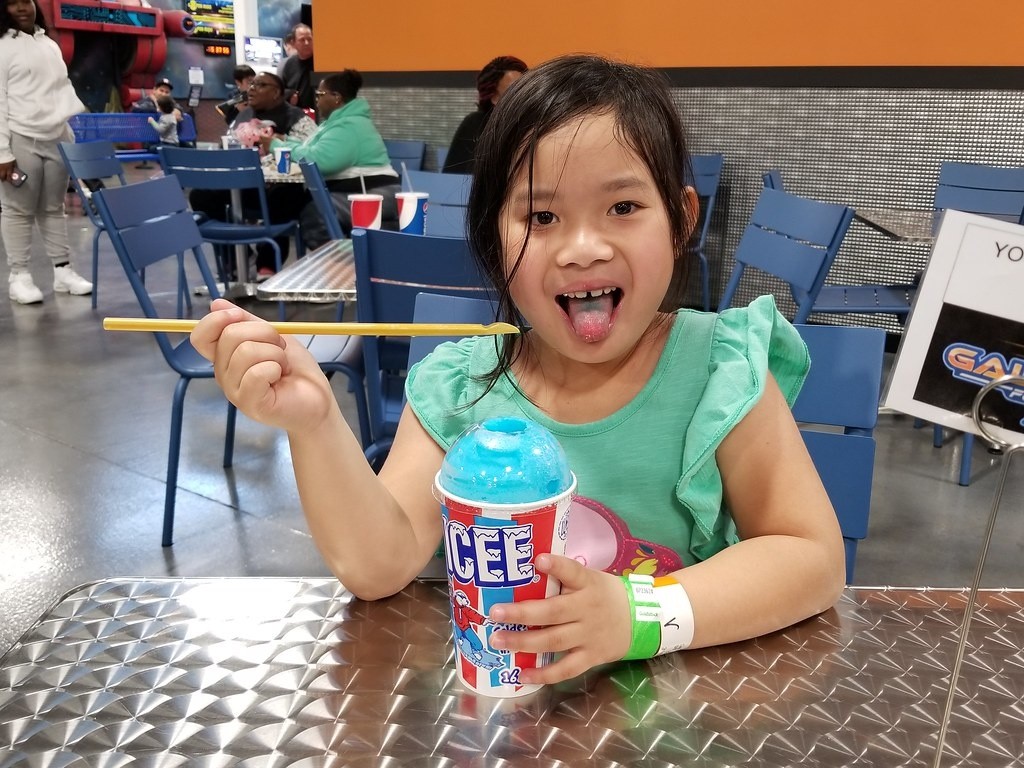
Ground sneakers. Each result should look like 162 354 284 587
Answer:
53 264 93 295
9 272 43 305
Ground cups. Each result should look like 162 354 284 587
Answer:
274 148 292 174
222 135 233 150
347 194 383 230
432 419 577 697
394 192 429 236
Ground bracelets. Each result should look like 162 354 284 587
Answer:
619 572 661 661
652 575 695 658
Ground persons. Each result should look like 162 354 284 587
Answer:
276 21 316 111
258 68 402 251
0 0 95 304
66 55 107 217
188 51 849 683
225 64 256 124
130 78 185 113
146 95 180 147
188 72 314 284
441 55 528 174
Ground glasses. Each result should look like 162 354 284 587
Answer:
248 82 279 89
314 90 336 98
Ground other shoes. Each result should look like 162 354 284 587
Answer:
83 198 98 215
256 268 275 281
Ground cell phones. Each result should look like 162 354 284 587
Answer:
9 166 28 187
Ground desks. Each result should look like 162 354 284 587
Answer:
854 209 1024 455
169 164 309 298
1 575 1024 768
258 236 358 302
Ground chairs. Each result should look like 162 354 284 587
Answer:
57 137 1023 586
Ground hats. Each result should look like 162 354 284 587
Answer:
156 78 174 91
